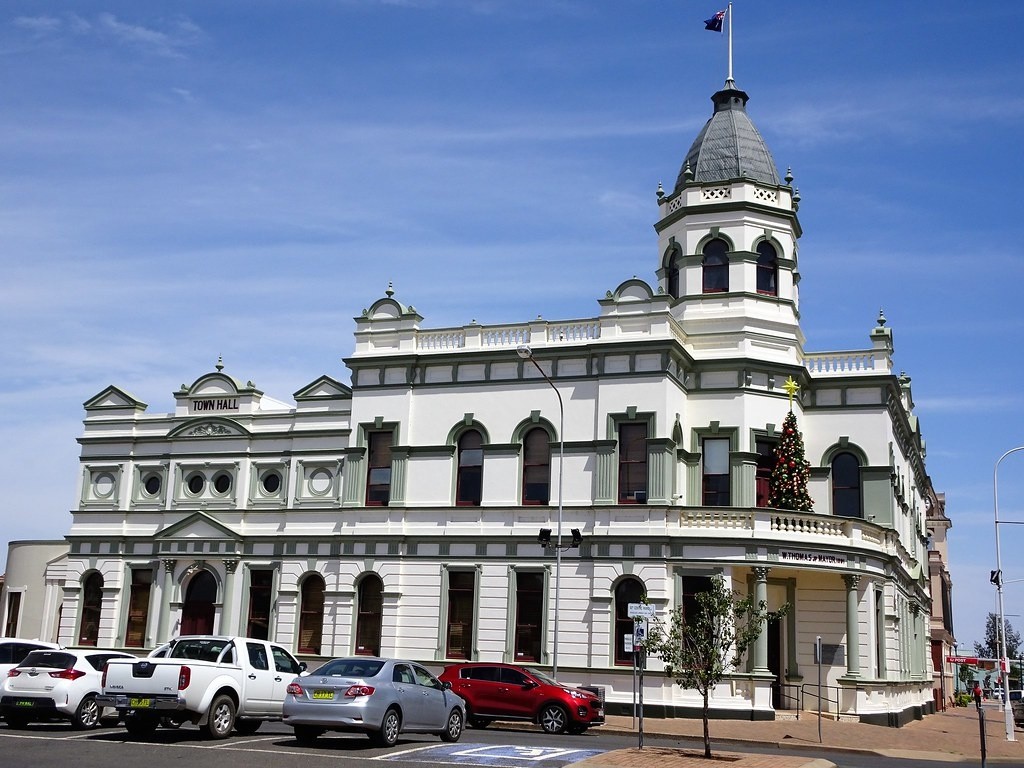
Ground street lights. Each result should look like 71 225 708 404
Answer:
516 343 564 682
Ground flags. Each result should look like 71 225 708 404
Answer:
703 9 726 32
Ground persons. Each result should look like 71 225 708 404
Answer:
972 682 982 712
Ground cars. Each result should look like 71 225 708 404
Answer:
0 649 142 731
0 637 69 686
282 655 467 748
994 688 1006 699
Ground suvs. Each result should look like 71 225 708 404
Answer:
437 661 606 734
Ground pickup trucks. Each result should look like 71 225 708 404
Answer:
93 636 309 739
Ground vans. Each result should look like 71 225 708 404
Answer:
1009 690 1024 709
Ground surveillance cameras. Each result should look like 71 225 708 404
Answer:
868 515 875 518
673 494 682 499
